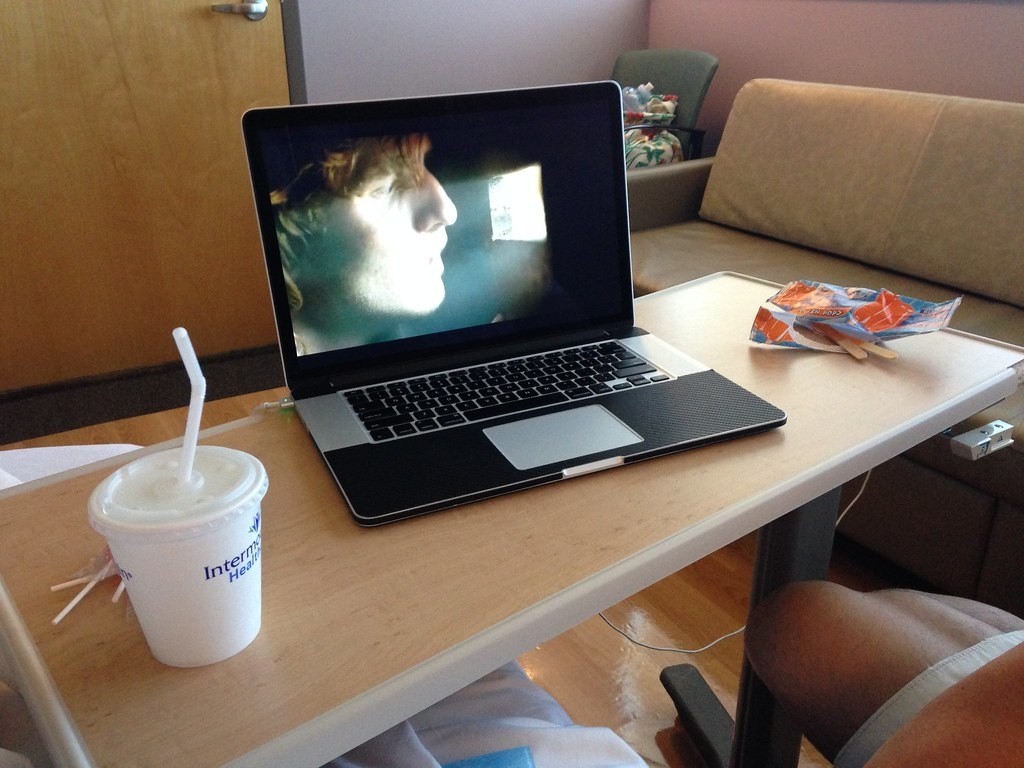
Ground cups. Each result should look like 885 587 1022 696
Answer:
88 444 269 667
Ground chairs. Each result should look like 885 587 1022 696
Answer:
612 49 718 167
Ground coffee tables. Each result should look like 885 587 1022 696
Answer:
3 269 1024 766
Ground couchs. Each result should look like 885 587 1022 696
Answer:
627 77 1024 620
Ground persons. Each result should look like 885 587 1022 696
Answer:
745 570 1024 767
265 113 459 356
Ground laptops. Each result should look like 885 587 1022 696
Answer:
240 77 788 531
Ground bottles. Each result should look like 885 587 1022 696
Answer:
622 82 654 113
